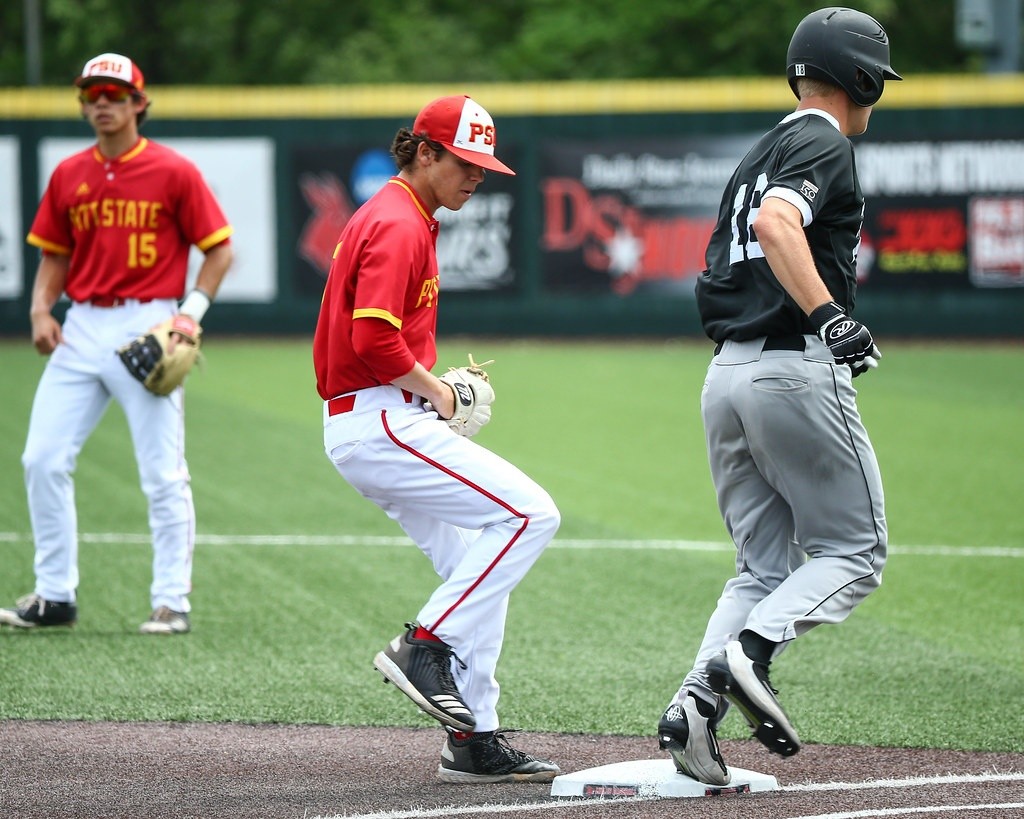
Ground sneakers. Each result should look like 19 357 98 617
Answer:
373 621 476 733
139 605 190 635
657 687 731 786
0 592 76 628
439 726 561 784
706 633 800 759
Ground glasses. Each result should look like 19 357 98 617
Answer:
81 83 135 104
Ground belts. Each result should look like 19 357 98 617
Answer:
714 335 806 351
328 388 412 416
91 295 153 307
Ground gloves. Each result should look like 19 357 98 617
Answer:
810 302 883 378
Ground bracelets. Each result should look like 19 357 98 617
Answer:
180 292 209 322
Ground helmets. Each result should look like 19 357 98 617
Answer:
787 7 904 107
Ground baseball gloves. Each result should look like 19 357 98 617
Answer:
429 351 498 438
115 313 203 407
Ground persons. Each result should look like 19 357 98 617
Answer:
657 7 902 786
313 95 560 779
1 54 235 634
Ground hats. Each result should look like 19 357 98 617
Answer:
413 95 516 177
76 53 143 94
